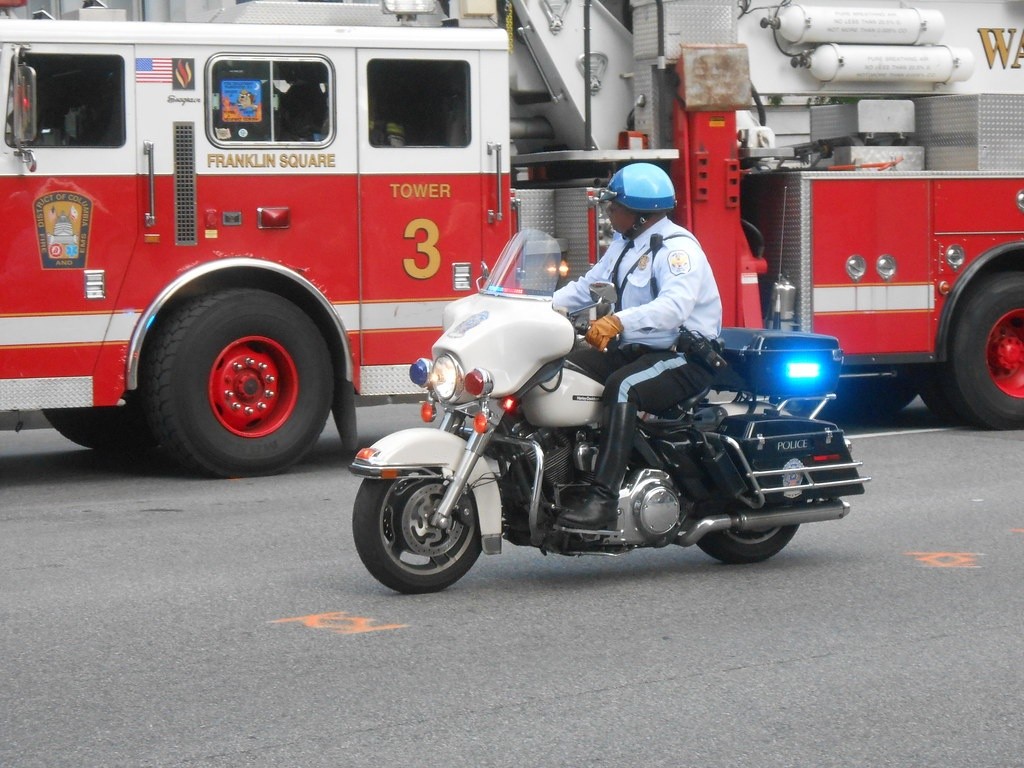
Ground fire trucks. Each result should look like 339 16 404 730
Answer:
0 0 1024 477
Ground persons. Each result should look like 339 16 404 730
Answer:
370 60 466 148
273 64 327 142
552 162 723 533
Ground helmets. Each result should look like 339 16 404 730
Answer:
598 162 677 212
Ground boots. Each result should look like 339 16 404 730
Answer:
556 402 639 530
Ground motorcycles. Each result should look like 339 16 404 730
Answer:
349 229 871 595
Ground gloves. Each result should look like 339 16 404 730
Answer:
587 315 623 352
